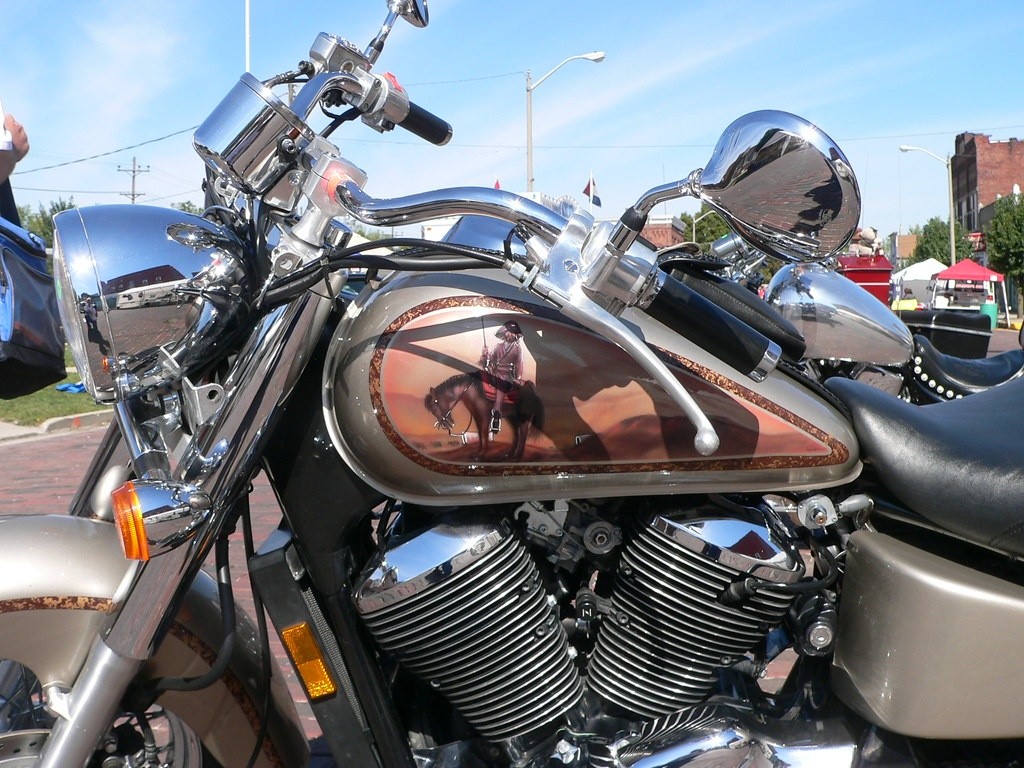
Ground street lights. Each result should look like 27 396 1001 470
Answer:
526 51 606 194
898 144 956 267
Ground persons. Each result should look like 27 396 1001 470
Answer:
80 297 98 330
904 288 914 299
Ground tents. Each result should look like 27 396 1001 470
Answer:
926 259 1015 329
890 257 953 310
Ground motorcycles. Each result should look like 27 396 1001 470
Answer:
1 0 1022 768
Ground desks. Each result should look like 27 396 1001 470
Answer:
931 306 981 311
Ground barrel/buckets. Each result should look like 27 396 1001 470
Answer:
979 303 998 329
